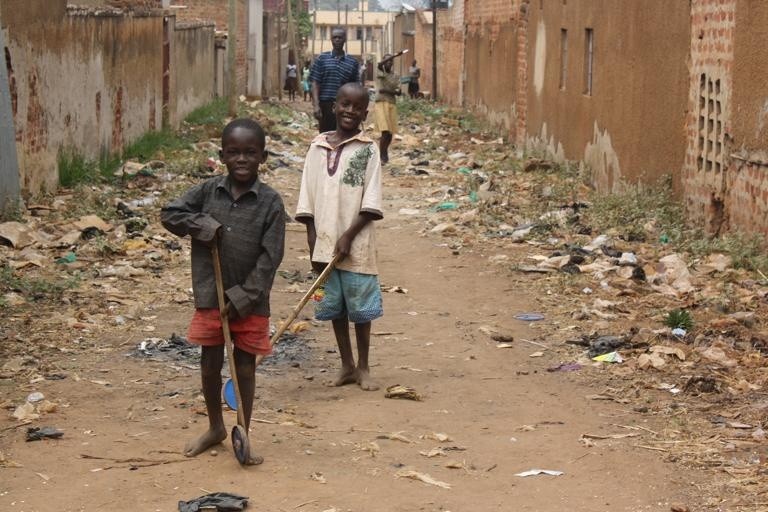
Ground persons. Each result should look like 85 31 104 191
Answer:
281 51 422 101
312 26 360 136
295 83 404 391
158 119 288 466
375 49 410 162
